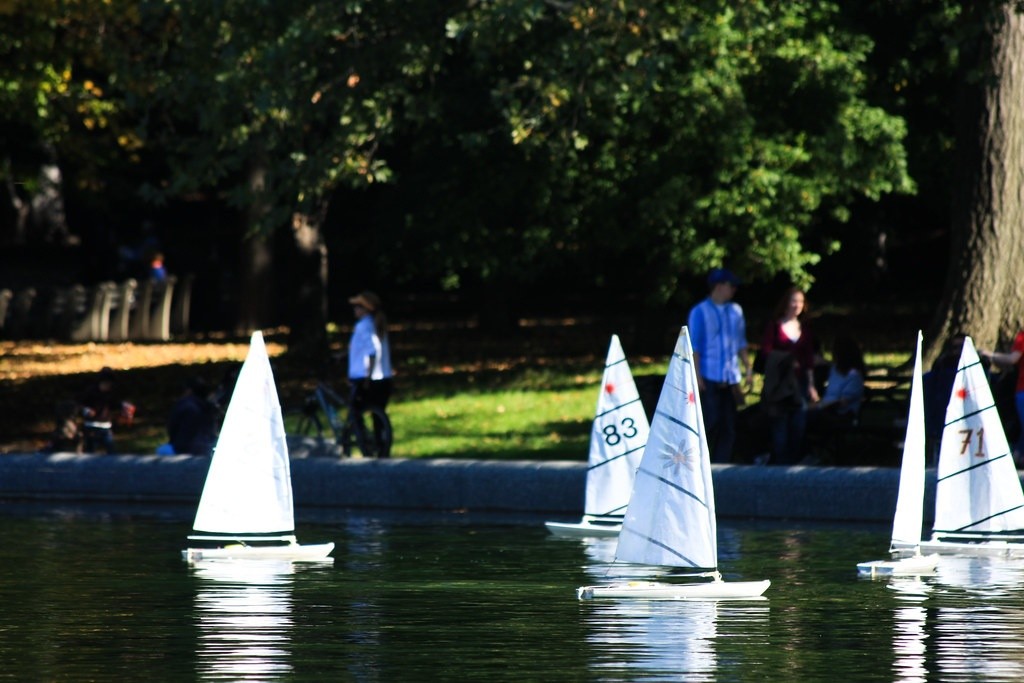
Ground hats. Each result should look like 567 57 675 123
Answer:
710 270 742 286
349 295 374 311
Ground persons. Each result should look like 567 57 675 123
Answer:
688 271 753 464
341 291 395 457
809 337 863 457
751 287 821 464
167 384 212 456
979 328 1024 465
43 376 136 454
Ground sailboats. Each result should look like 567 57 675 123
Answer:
179 328 337 568
573 324 773 605
544 332 651 539
855 329 1024 588
